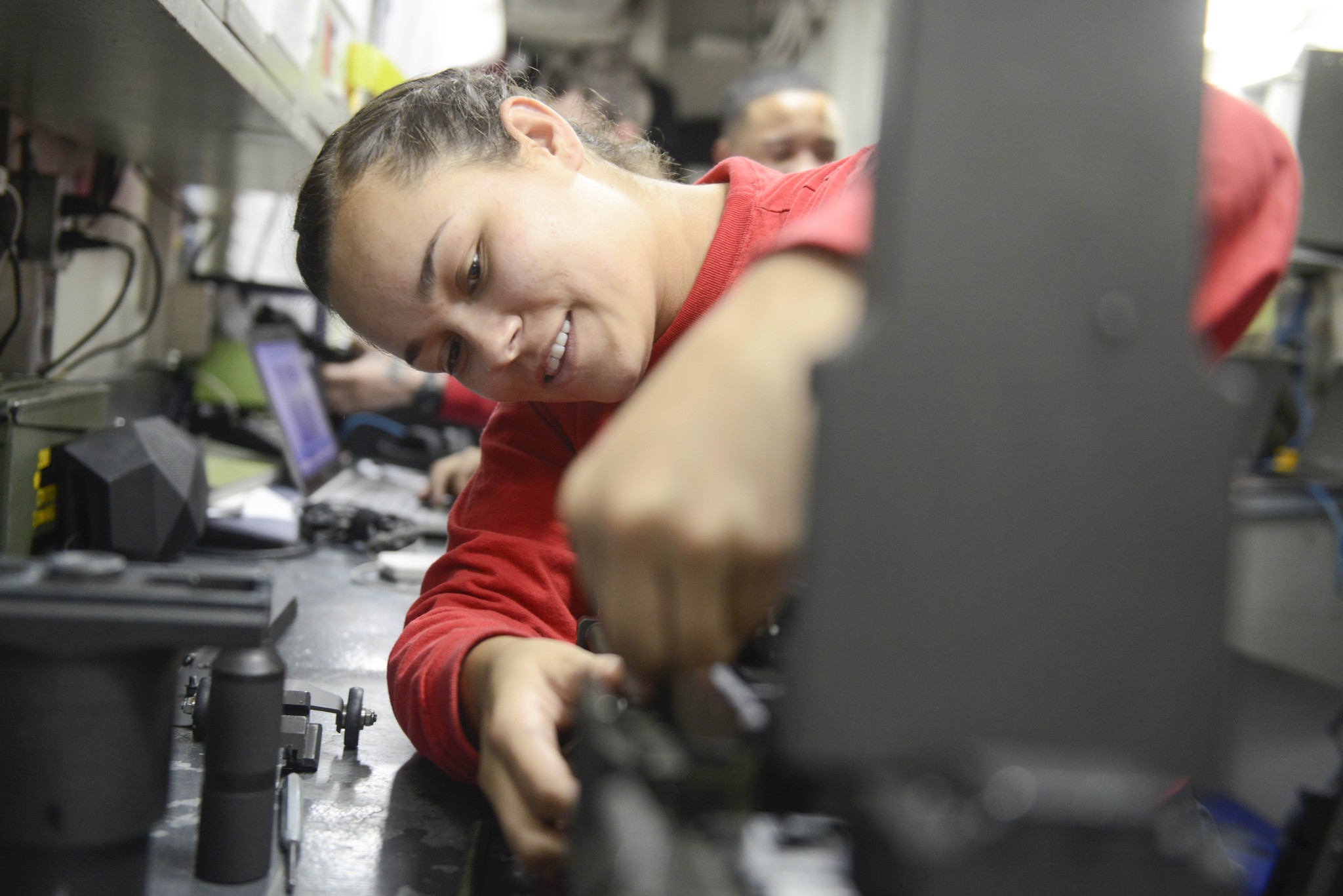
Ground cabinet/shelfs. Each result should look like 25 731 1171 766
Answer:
0 1 482 199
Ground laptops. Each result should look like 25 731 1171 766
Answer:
249 325 458 535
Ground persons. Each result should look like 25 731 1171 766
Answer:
322 46 658 507
712 64 845 176
295 59 1302 896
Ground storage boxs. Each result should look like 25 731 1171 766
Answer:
247 1 507 124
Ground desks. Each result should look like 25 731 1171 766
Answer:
0 533 466 896
